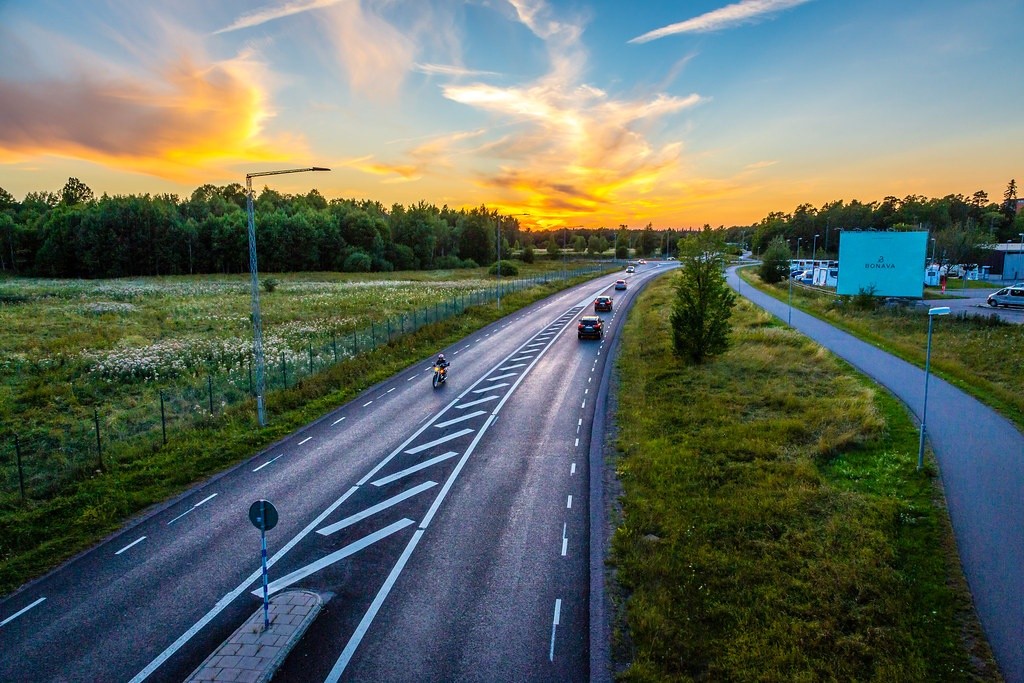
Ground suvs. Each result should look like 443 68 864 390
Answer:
578 316 605 340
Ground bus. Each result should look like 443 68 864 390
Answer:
793 259 834 271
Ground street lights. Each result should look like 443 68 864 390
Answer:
930 238 936 292
243 167 333 425
915 307 951 473
494 213 529 309
797 237 803 275
1004 240 1012 283
812 234 820 286
757 246 760 263
1018 232 1024 283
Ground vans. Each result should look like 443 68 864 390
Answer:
987 287 1024 307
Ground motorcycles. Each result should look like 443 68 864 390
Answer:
431 361 451 388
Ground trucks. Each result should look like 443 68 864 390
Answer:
813 267 838 287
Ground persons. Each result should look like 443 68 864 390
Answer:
433 354 446 378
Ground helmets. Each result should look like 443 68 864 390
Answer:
439 354 444 360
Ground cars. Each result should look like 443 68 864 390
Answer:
639 259 646 263
625 266 635 272
667 257 674 260
789 269 812 286
594 296 613 311
614 280 628 289
931 258 991 281
